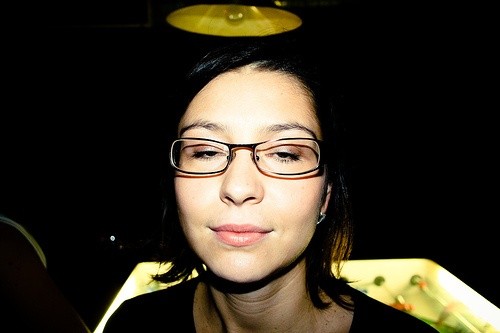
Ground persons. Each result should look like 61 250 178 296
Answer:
103 43 438 333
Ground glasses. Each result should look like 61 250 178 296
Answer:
169 138 327 176
